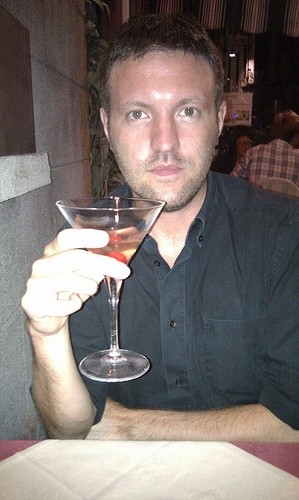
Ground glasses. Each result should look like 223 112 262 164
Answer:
238 141 251 144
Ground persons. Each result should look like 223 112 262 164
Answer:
20 11 299 441
218 134 257 176
230 108 299 187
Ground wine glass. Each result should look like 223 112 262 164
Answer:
56 197 166 382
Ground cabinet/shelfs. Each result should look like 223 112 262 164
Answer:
206 24 298 153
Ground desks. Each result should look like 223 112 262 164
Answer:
0 441 298 500
